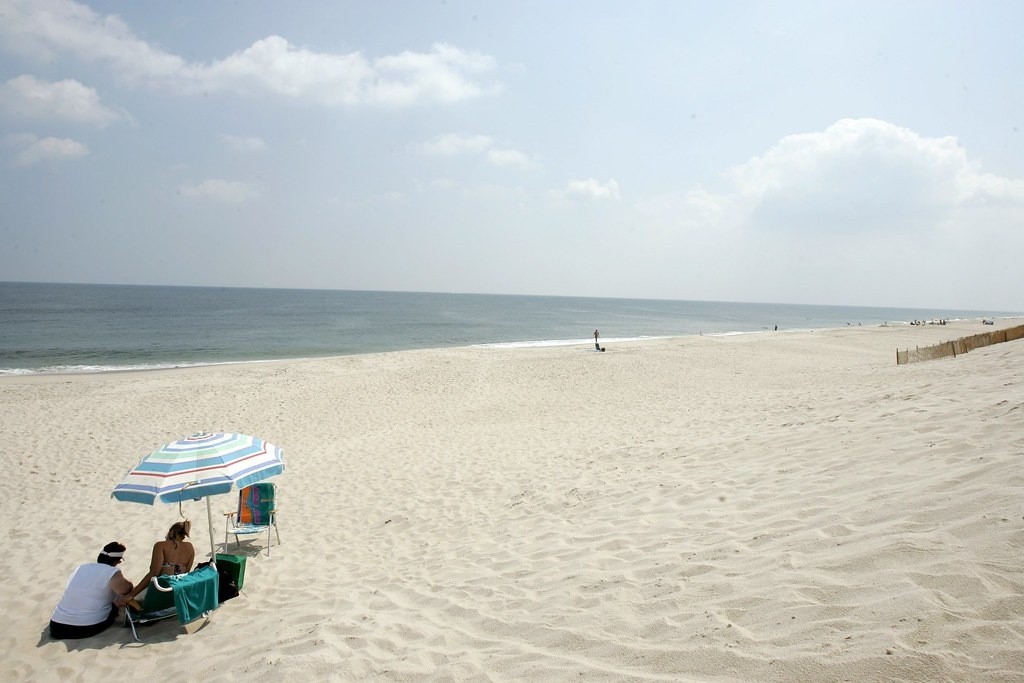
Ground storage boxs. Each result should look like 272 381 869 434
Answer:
209 553 247 591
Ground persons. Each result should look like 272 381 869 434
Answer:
593 329 600 342
983 319 993 325
910 318 945 326
113 520 195 608
50 541 133 639
775 323 778 331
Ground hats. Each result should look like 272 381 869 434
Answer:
100 550 125 561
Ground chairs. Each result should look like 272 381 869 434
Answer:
111 562 219 641
224 482 281 557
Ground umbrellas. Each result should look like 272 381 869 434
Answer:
109 431 287 565
